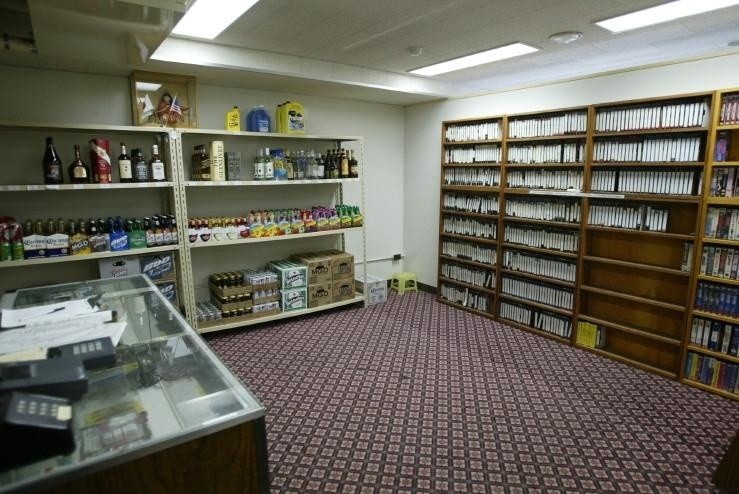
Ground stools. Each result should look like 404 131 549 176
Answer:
391 271 418 296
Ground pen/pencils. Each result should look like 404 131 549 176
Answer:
19 307 65 322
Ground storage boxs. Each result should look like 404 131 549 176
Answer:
98 251 180 314
355 273 388 305
267 249 355 312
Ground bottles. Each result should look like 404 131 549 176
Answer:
313 204 360 218
192 145 210 181
246 105 270 132
1 222 24 260
248 207 313 223
118 139 166 181
189 215 249 228
225 104 240 130
24 216 143 236
42 137 63 185
144 212 178 247
68 144 90 184
254 145 359 180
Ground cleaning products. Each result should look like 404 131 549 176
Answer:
226 105 240 131
277 101 305 135
245 105 270 133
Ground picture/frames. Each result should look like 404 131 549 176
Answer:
129 69 199 130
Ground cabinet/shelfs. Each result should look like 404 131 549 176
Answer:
679 88 738 398
175 127 369 337
0 274 273 493
573 92 717 384
0 120 192 348
506 105 591 349
439 114 507 320
